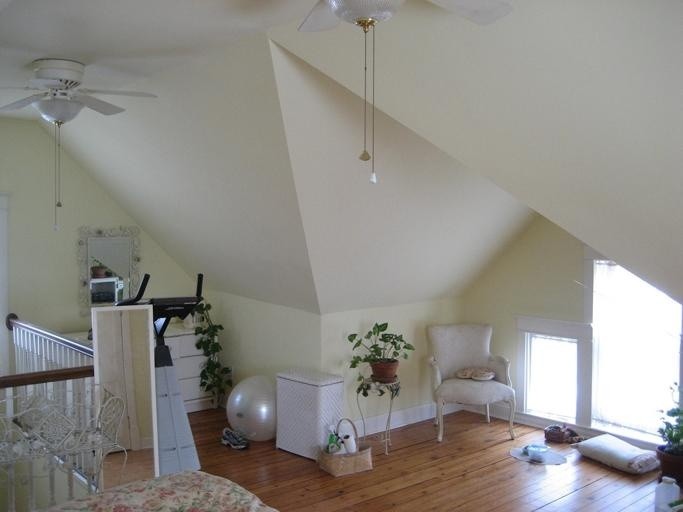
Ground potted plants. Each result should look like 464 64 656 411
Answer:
342 321 413 382
653 385 683 483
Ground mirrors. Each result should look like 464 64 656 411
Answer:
76 226 142 318
92 304 159 489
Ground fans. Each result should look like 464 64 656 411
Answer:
0 58 158 114
293 0 515 34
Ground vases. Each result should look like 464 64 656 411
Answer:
91 266 108 278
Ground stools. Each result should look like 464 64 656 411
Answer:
275 368 345 463
356 379 402 457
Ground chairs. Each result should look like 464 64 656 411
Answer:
423 320 516 444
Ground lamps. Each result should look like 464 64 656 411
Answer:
328 1 402 33
31 90 85 127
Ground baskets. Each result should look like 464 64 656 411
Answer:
318 418 373 477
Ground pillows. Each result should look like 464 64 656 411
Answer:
457 365 497 382
572 434 659 478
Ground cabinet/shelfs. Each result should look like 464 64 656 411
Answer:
162 320 219 413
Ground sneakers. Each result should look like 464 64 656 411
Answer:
220 429 247 449
